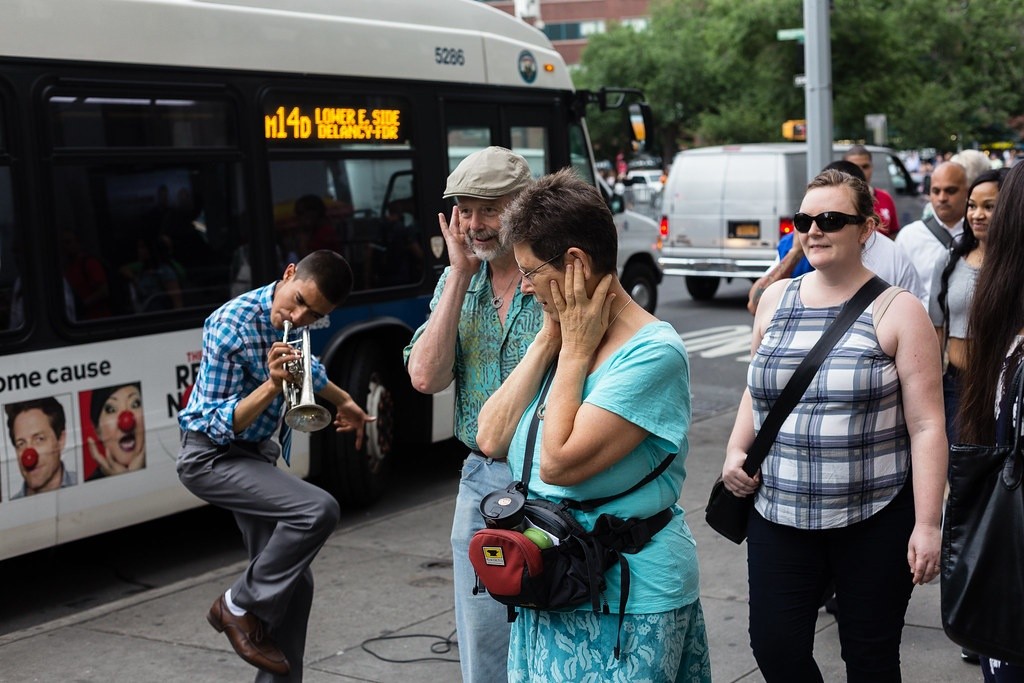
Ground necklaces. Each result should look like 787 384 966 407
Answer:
489 267 521 309
537 300 631 420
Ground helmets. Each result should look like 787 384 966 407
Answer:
443 145 532 203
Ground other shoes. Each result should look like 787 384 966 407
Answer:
962 649 976 666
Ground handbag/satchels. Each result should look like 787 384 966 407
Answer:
705 475 753 545
937 362 1024 661
467 481 674 614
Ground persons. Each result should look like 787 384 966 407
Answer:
5 397 76 498
176 251 376 683
747 147 928 316
84 381 146 482
922 149 1024 683
895 149 989 292
403 146 544 683
56 194 425 324
476 169 713 682
721 169 949 683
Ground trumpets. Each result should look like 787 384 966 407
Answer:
281 320 331 432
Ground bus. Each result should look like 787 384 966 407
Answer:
0 0 656 566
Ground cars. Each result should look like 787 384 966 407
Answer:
625 169 667 210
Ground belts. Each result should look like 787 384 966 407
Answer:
471 448 507 462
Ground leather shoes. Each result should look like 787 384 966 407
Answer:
206 588 290 676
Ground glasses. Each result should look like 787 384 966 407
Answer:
795 211 865 236
518 250 570 281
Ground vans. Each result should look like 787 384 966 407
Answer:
655 140 932 296
329 145 666 318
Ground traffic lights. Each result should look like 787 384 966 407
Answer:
783 120 808 141
